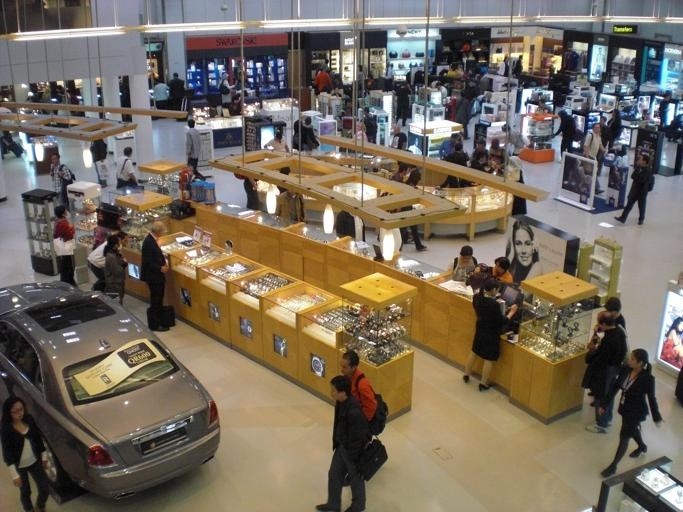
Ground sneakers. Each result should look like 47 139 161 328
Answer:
629 446 647 457
585 423 609 433
594 189 604 194
415 245 424 249
316 503 340 511
343 506 365 512
614 216 626 223
600 464 616 476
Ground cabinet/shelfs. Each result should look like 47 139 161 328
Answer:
594 456 683 511
17 86 682 427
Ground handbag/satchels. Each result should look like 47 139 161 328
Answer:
146 305 175 327
356 439 387 481
115 178 137 189
647 175 654 192
368 394 388 436
52 237 76 256
572 129 584 142
579 136 584 154
40 448 56 482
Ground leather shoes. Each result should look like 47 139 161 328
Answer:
151 324 168 331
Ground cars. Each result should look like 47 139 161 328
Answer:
0 279 221 503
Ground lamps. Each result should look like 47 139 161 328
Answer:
0 1 136 170
208 2 466 259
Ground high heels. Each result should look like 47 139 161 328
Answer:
478 383 489 391
462 374 469 383
